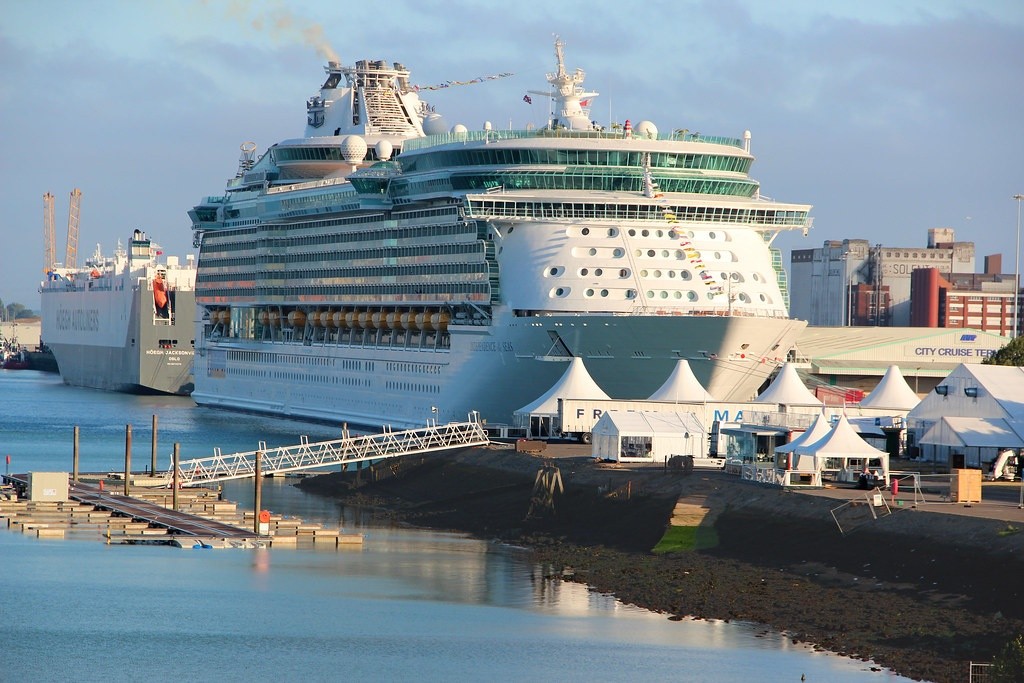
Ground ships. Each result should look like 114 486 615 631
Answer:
187 32 817 445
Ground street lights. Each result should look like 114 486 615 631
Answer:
1012 194 1024 340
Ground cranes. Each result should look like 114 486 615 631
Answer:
41 188 82 281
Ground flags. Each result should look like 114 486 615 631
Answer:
580 98 593 107
523 96 532 104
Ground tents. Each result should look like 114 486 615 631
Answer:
514 358 1024 489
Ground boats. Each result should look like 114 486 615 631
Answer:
319 312 334 328
358 311 374 330
430 312 449 330
209 311 218 323
345 312 360 330
385 312 402 331
307 310 322 327
288 310 307 327
415 312 433 331
400 312 418 330
332 310 345 328
217 311 230 324
371 311 388 331
258 311 270 326
37 227 197 396
153 276 167 309
269 311 281 328
